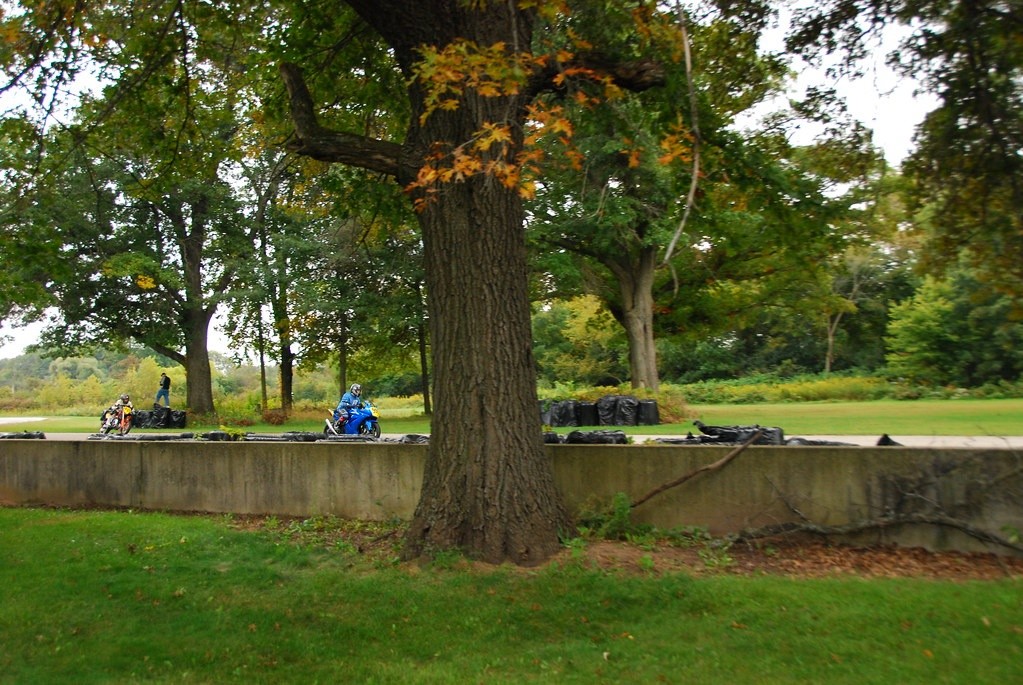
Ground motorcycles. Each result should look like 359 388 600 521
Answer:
100 404 136 434
324 400 381 438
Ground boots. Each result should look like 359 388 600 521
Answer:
333 419 340 428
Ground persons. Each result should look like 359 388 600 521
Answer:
156 373 170 406
104 394 135 427
333 383 362 432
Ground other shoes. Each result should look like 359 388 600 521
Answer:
164 406 169 408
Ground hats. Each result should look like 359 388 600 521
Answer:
161 373 165 376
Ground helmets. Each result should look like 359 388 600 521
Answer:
120 394 129 404
350 384 361 397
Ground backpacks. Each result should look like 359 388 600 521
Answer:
163 376 170 389
